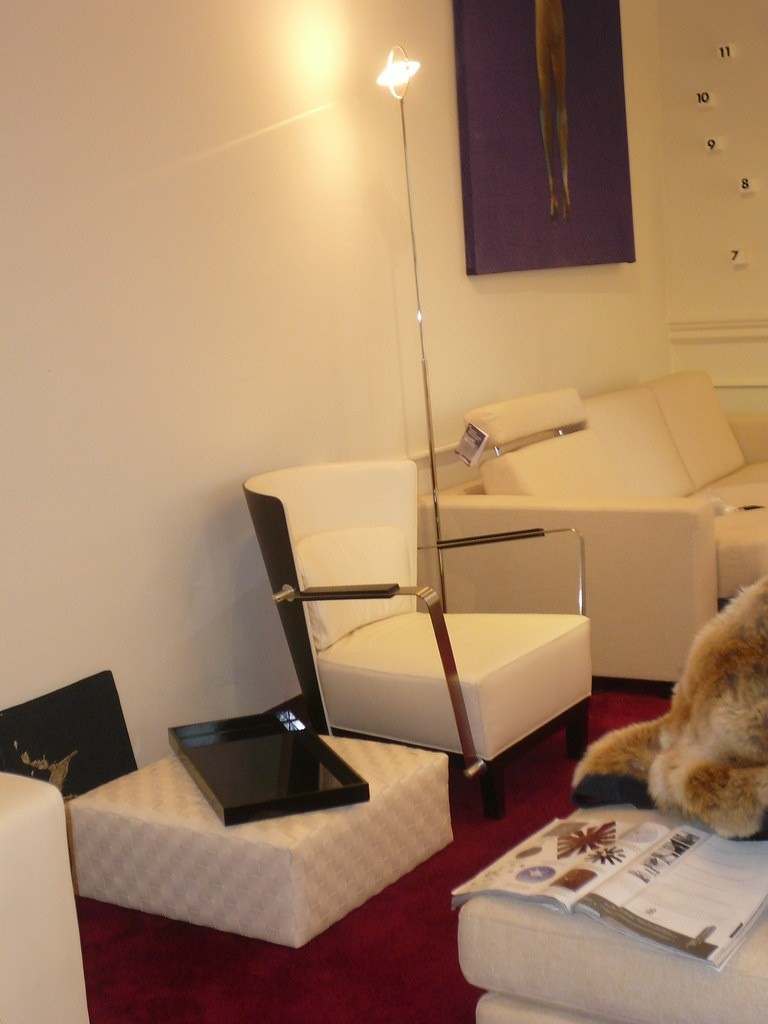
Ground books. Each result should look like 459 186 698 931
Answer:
449 814 768 970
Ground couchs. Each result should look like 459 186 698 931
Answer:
422 367 768 685
455 805 768 1024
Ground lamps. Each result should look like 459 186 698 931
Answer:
376 44 447 614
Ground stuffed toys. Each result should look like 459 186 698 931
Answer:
569 576 767 838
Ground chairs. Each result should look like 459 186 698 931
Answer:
242 458 595 821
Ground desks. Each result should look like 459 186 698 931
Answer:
65 730 458 949
